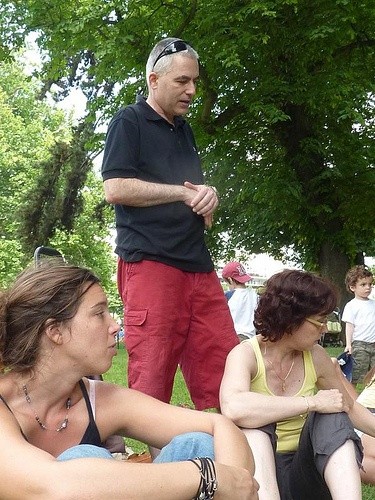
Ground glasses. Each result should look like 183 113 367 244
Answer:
303 317 328 333
153 40 194 69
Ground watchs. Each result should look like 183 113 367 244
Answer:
209 186 220 196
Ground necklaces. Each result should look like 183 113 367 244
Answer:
22 384 71 433
260 351 295 391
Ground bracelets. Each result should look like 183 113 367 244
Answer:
187 455 217 500
300 395 310 420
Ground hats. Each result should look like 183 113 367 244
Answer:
337 351 358 382
222 261 252 284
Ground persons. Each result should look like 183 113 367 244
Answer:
221 262 261 344
0 256 260 500
342 265 375 385
100 38 242 464
219 268 375 500
